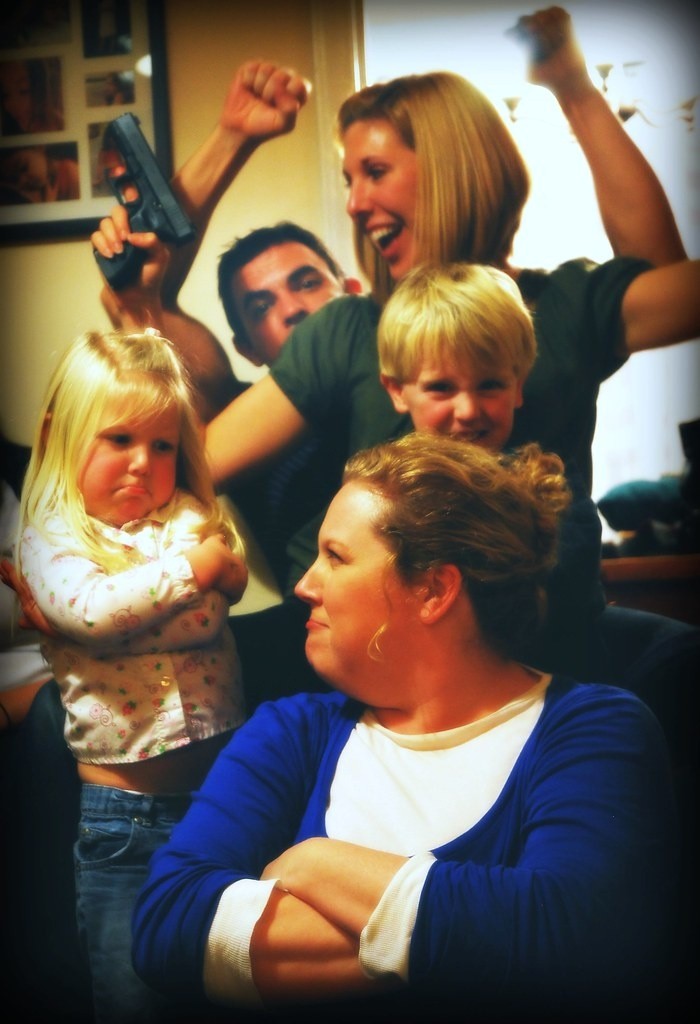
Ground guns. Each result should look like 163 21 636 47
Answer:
86 112 195 292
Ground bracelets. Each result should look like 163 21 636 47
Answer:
0 704 11 729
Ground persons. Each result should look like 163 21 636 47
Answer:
0 6 700 1024
14 327 247 1023
133 434 691 1024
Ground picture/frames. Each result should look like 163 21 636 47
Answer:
0 0 174 250
